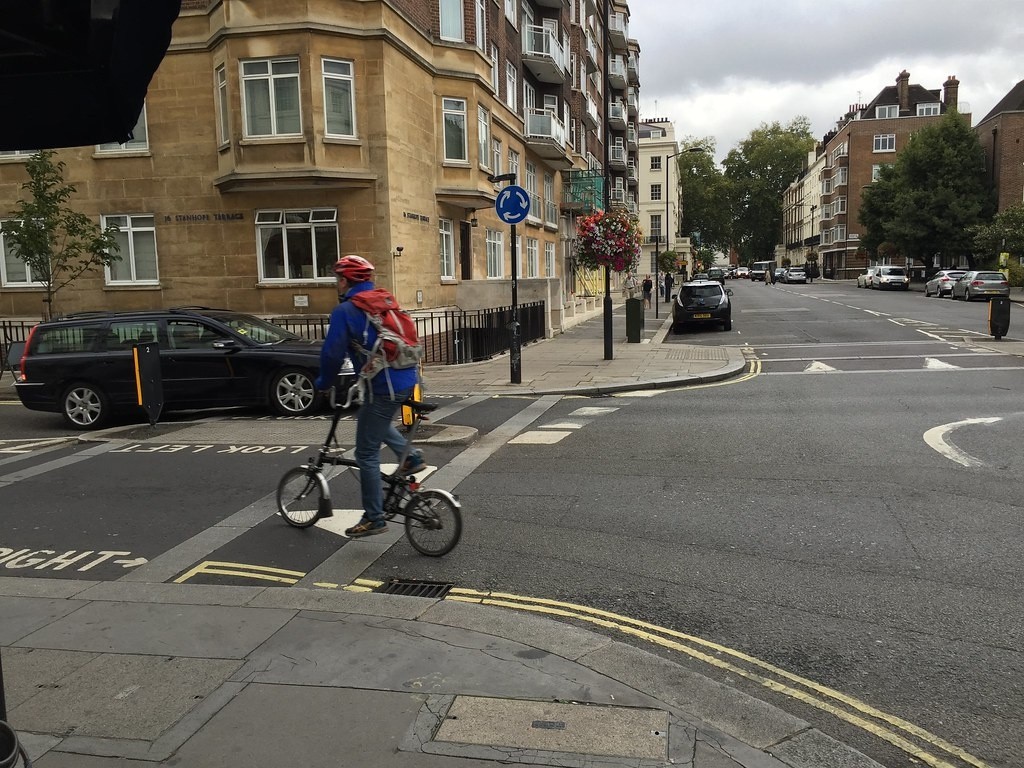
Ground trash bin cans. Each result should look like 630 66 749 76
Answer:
626 298 643 342
132 342 163 425
988 297 1010 340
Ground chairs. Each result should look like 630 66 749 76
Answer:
168 324 209 349
108 329 154 349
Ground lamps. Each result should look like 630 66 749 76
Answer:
470 208 478 227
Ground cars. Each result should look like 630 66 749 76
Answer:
925 269 968 298
690 265 751 285
857 266 910 291
775 267 807 284
951 270 1010 301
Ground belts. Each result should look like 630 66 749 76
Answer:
628 288 632 289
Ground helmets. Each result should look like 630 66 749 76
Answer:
334 253 375 282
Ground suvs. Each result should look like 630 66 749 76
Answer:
671 281 734 333
14 307 359 432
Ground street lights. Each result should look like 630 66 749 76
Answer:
773 218 788 264
664 147 704 303
794 203 813 283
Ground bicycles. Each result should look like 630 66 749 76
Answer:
278 381 463 557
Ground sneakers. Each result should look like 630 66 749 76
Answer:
399 455 426 475
344 519 388 536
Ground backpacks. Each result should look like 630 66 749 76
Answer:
346 287 422 380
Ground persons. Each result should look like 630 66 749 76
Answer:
624 272 638 298
765 268 775 286
659 272 665 297
616 98 623 106
316 255 426 537
642 274 652 309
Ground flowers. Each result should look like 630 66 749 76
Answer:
573 206 643 276
658 250 678 273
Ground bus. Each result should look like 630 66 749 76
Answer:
750 260 777 281
